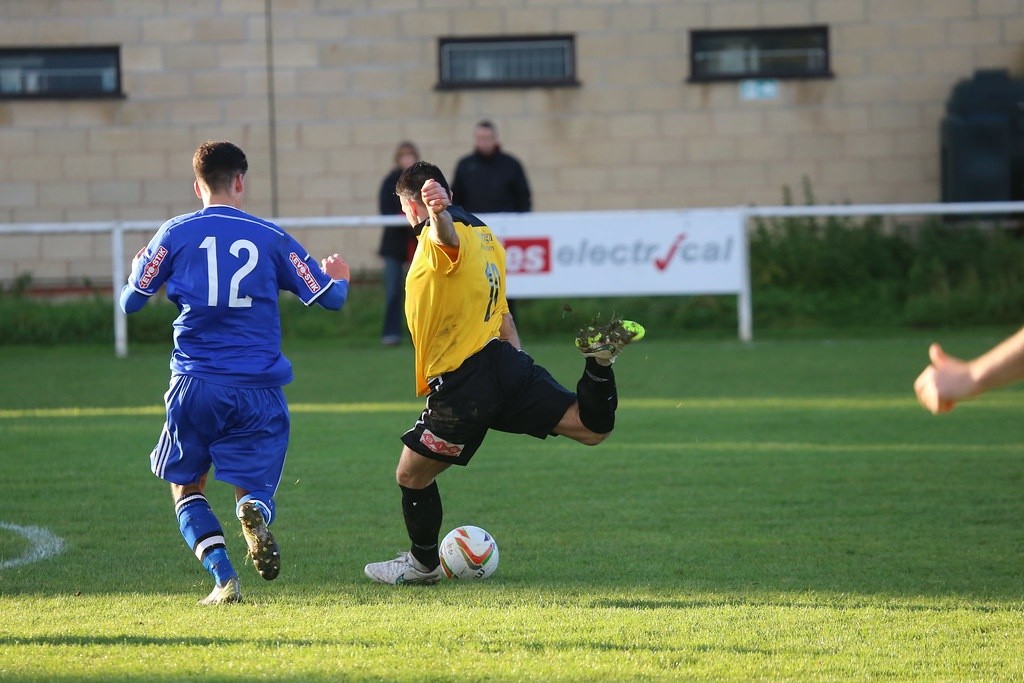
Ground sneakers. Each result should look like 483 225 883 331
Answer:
364 551 442 585
574 320 646 364
197 578 242 605
238 502 281 581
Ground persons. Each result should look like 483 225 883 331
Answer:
914 328 1024 412
450 121 532 331
119 141 349 605
366 162 646 585
379 143 419 344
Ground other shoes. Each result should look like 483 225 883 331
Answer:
382 335 400 344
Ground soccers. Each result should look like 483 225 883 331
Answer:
439 524 500 580
